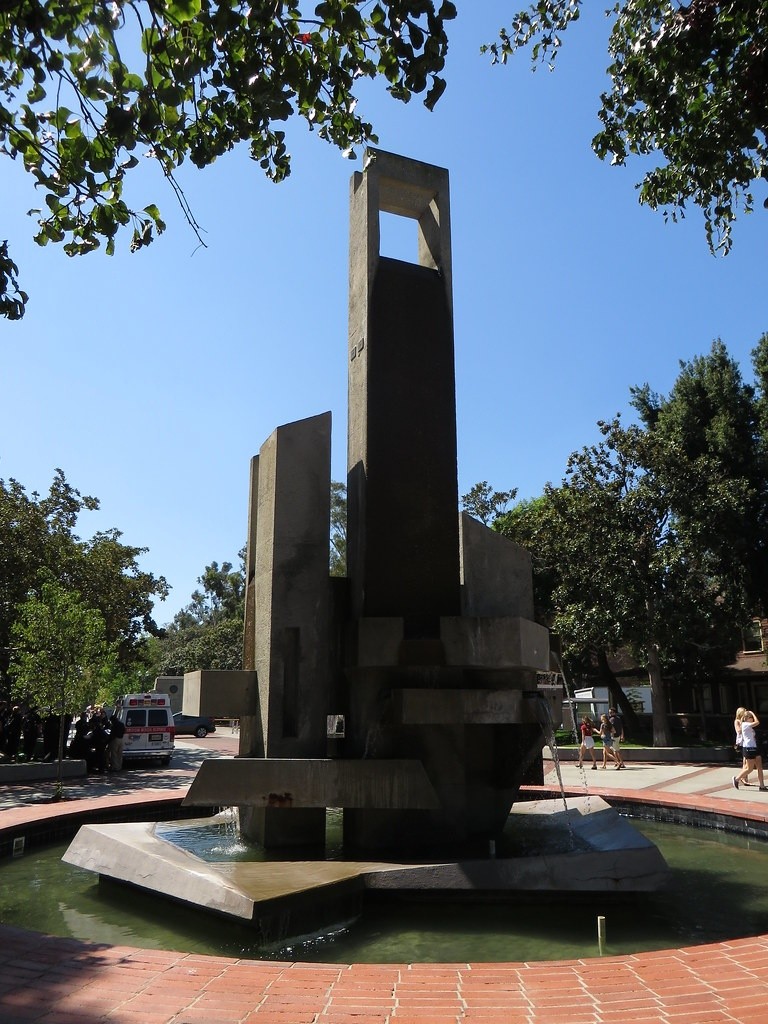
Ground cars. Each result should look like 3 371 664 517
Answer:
171 711 217 739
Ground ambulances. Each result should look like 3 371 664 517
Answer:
115 693 175 766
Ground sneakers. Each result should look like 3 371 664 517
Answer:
732 777 740 790
759 787 768 792
740 778 750 786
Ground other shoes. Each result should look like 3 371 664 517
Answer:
591 766 597 769
575 764 583 767
600 766 606 769
613 763 626 770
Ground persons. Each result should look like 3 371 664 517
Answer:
0 700 125 773
732 707 768 792
576 707 625 770
